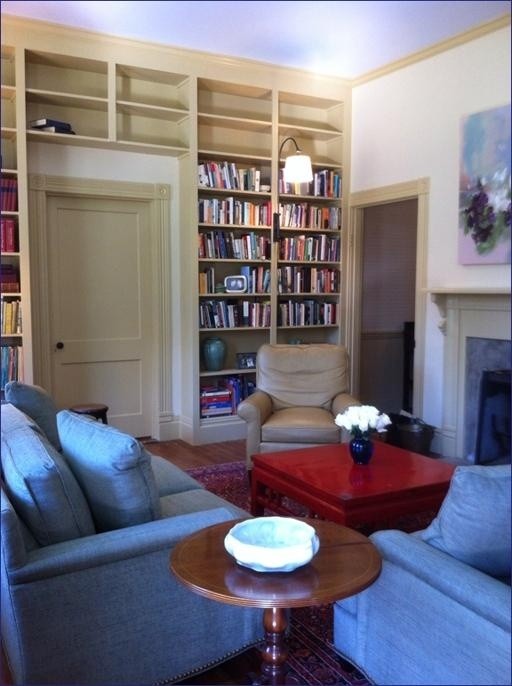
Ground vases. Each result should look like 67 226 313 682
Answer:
201 336 226 372
349 433 373 464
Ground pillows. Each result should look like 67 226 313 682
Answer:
417 463 511 577
5 381 159 532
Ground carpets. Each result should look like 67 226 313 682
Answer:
184 458 439 686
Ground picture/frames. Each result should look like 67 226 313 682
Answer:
236 351 257 370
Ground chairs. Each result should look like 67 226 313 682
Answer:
235 342 363 485
331 531 512 684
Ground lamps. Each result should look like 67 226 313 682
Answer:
273 137 314 244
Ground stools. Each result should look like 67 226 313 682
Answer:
70 402 108 424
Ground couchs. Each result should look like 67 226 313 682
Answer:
1 382 293 684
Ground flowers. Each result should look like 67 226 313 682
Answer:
332 403 394 439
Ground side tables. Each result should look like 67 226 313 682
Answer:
169 516 384 685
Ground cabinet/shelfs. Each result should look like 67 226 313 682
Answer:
177 74 351 448
0 43 190 384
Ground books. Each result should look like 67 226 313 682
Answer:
198 161 342 418
0 172 24 391
30 118 76 135
